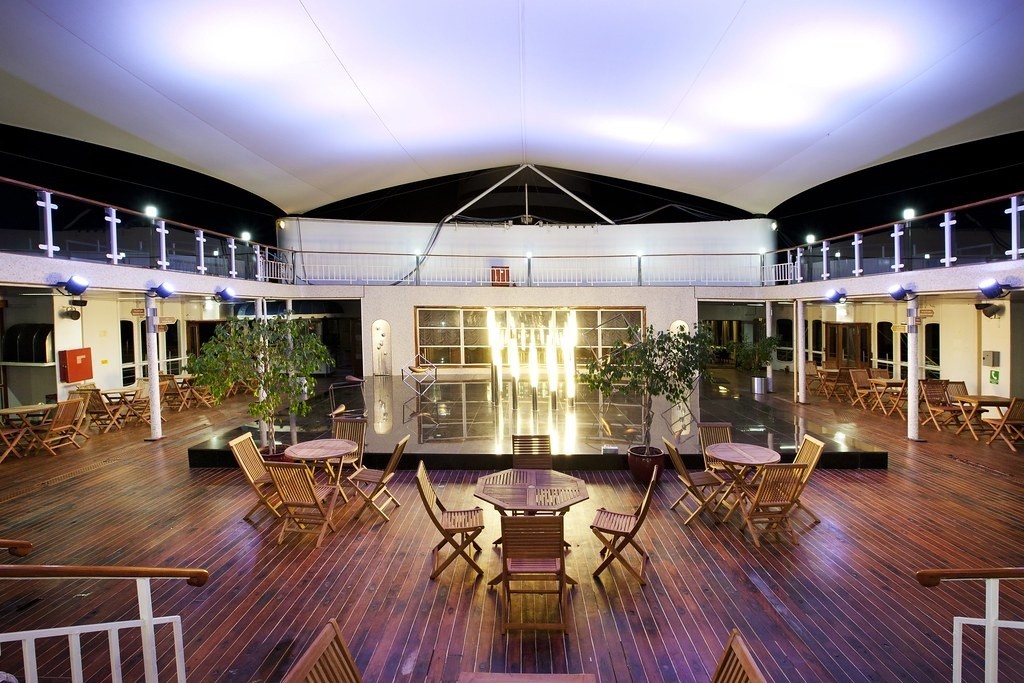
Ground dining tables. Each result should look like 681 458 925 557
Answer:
705 441 782 525
816 369 853 396
174 373 214 412
705 442 782 524
952 393 1017 444
0 402 60 452
982 396 1024 455
102 386 144 421
284 436 359 508
473 467 590 549
868 378 907 415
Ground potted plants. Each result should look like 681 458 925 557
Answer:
728 335 779 395
580 319 714 482
181 314 335 467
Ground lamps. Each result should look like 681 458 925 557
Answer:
978 277 1013 299
149 282 174 299
57 274 89 297
888 283 919 303
826 289 848 305
217 287 235 302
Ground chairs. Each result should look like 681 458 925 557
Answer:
325 416 369 499
821 360 840 370
825 366 856 403
870 367 893 408
803 360 823 393
30 397 85 456
886 378 927 422
920 379 963 431
130 380 171 425
76 388 124 432
76 382 98 389
347 433 412 524
943 380 991 430
771 433 826 530
590 463 660 587
696 421 752 506
159 374 191 412
660 436 724 527
738 461 810 548
415 460 488 580
850 368 880 409
227 430 297 522
499 514 570 638
0 428 28 464
51 390 92 445
263 460 339 549
511 433 554 470
124 377 152 423
